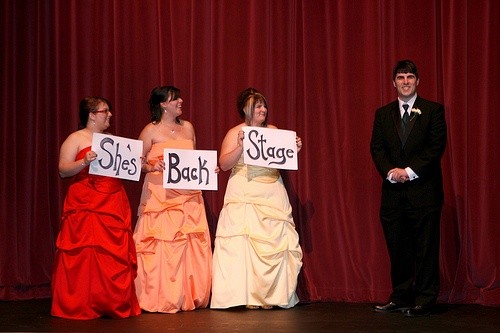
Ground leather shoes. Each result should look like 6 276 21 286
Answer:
403 303 436 317
374 302 410 313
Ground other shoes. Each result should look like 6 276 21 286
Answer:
246 305 260 309
260 306 273 309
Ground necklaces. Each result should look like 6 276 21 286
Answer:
164 120 178 133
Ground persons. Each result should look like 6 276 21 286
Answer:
370 59 448 318
209 88 304 311
132 85 213 314
50 96 145 321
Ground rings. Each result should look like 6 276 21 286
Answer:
237 133 240 135
401 180 404 184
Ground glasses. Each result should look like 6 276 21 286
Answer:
96 109 110 113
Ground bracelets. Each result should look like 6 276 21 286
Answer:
82 158 87 167
237 144 241 148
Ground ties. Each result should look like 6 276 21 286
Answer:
402 104 410 130
